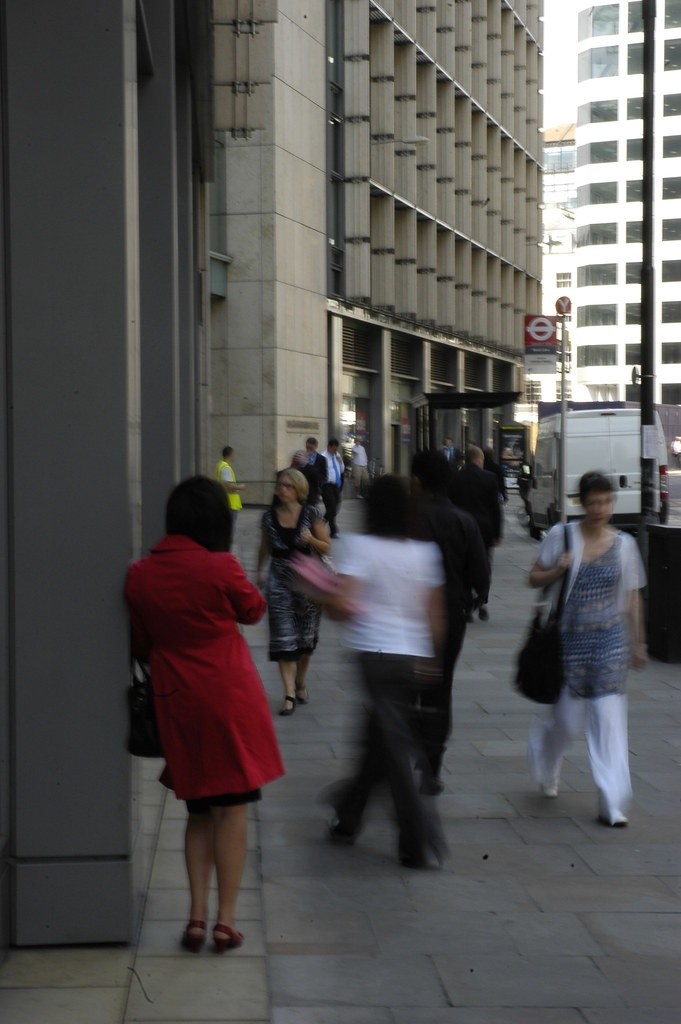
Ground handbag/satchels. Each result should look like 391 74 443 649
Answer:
515 613 567 705
127 657 164 757
310 546 338 576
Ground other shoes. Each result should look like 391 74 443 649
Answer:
324 816 359 845
279 696 296 716
478 602 489 622
295 680 309 704
598 807 627 828
539 781 559 797
420 773 442 796
330 533 342 539
398 828 449 870
464 606 474 622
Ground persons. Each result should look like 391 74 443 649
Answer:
527 470 648 827
123 475 286 959
256 468 332 716
275 435 509 871
216 446 247 552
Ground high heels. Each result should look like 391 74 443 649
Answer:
182 921 206 954
213 923 244 954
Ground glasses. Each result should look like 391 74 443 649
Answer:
277 482 296 490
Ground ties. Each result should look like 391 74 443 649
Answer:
332 456 341 488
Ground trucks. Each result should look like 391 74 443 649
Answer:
520 409 670 541
